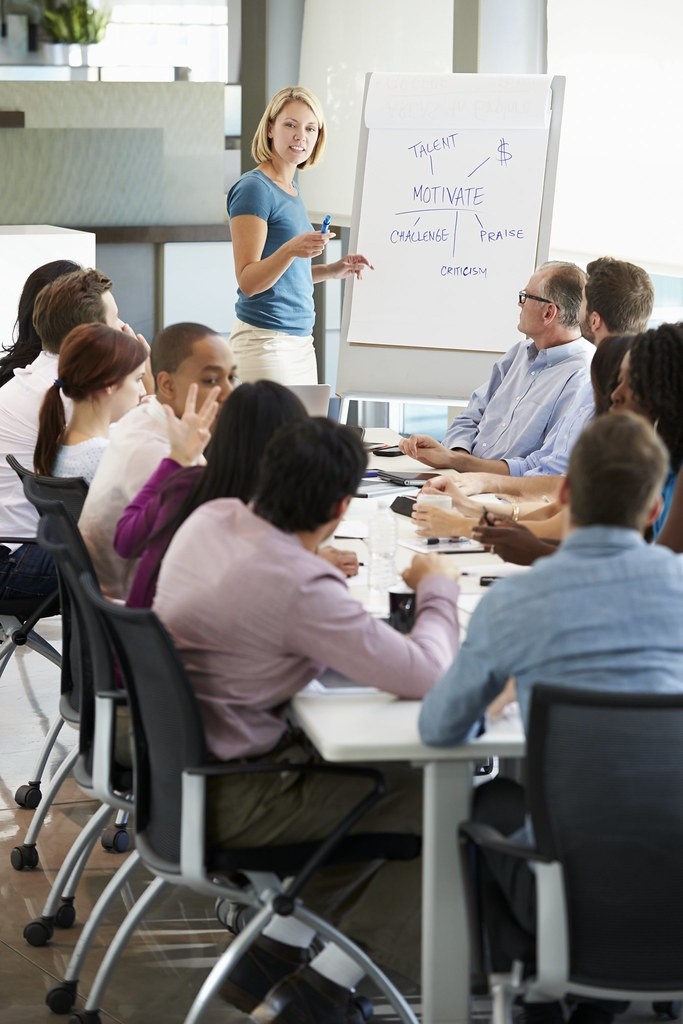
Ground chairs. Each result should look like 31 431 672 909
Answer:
459 682 683 1024
0 453 426 1024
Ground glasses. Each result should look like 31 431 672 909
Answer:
519 289 560 310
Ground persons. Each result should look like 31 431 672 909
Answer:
1 260 682 1024
227 86 374 386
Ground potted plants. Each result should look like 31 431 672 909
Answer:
35 0 112 67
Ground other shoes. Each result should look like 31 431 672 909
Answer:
248 962 367 1024
219 931 315 1014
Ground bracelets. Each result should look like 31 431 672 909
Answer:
511 503 519 521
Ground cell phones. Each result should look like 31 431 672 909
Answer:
480 576 501 585
372 446 404 457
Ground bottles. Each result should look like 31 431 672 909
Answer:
368 502 398 591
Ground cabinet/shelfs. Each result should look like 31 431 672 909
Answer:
0 224 96 360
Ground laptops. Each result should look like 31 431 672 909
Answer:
284 384 332 418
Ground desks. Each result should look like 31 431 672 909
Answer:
289 427 543 1024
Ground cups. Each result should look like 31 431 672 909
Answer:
417 495 452 538
389 587 416 635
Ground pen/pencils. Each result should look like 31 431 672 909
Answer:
360 472 378 478
321 215 332 233
358 561 367 566
420 537 469 545
